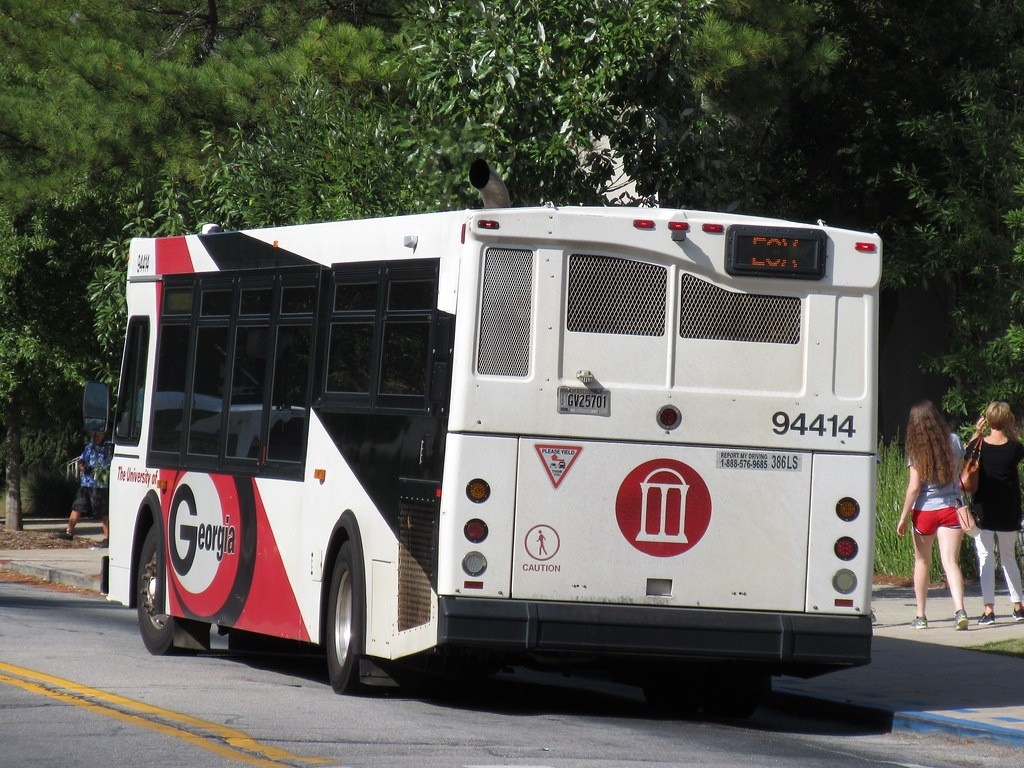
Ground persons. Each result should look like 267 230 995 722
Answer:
895 398 1024 630
56 433 108 548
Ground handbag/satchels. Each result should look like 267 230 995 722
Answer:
958 435 984 492
955 474 980 537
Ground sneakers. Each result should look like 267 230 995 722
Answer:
976 613 994 624
56 532 73 541
910 616 928 629
1012 608 1024 621
871 611 878 626
99 539 109 548
952 611 970 630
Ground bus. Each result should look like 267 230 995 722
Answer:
99 203 884 697
